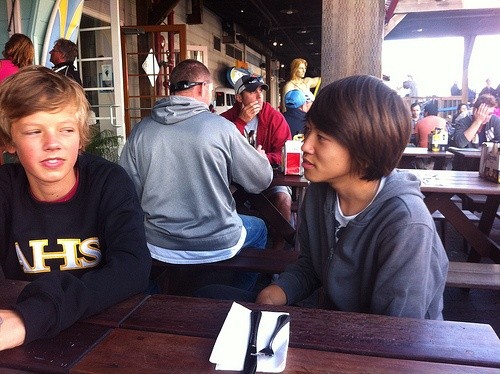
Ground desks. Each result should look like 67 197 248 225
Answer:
449 145 482 167
0 275 500 374
401 145 454 165
272 166 500 219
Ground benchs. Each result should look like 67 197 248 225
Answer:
438 260 499 303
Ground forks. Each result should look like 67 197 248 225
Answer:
250 314 293 357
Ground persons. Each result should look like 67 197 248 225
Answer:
448 78 500 171
411 101 449 148
282 58 321 138
49 39 82 86
256 73 449 321
0 64 153 352
117 60 274 266
220 75 293 225
0 33 34 82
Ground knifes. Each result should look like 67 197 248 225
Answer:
243 309 262 374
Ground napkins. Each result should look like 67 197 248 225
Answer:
209 299 292 374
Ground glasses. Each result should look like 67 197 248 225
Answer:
169 80 202 91
237 77 264 94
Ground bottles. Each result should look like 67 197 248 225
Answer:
491 140 499 155
249 130 257 150
432 127 446 152
297 130 304 142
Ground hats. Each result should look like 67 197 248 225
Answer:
234 75 269 95
285 89 305 108
